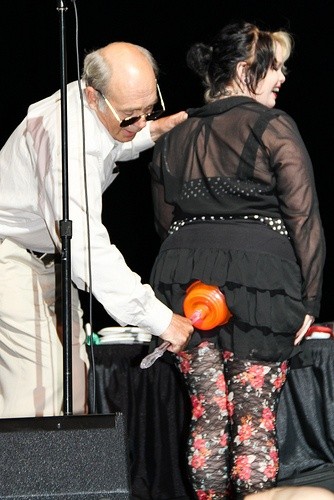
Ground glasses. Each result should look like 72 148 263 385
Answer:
95 79 165 127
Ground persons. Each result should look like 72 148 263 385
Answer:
150 23 326 500
0 42 194 418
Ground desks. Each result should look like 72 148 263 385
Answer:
88 322 334 500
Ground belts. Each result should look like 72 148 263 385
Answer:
27 249 55 263
